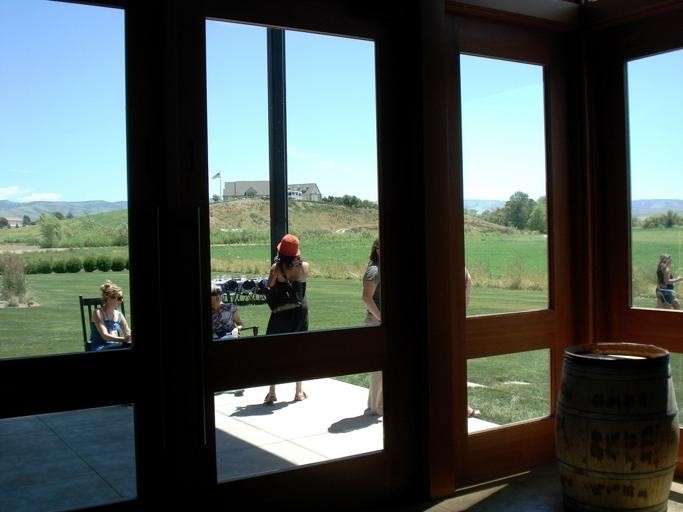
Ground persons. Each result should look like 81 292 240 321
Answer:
211 285 243 341
263 234 309 402
89 281 134 407
656 253 683 310
361 238 384 415
465 267 482 417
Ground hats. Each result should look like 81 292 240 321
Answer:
277 234 301 257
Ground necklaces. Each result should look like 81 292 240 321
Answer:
104 309 116 329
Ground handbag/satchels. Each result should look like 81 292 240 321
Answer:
264 262 303 320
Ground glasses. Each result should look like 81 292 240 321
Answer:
211 291 223 296
109 296 123 301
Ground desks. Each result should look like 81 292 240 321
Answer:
211 277 263 295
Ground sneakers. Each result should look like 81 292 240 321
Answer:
265 393 278 402
296 390 309 401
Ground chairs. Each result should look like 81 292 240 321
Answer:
222 276 268 304
79 284 259 352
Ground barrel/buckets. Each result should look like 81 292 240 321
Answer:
553 343 679 512
